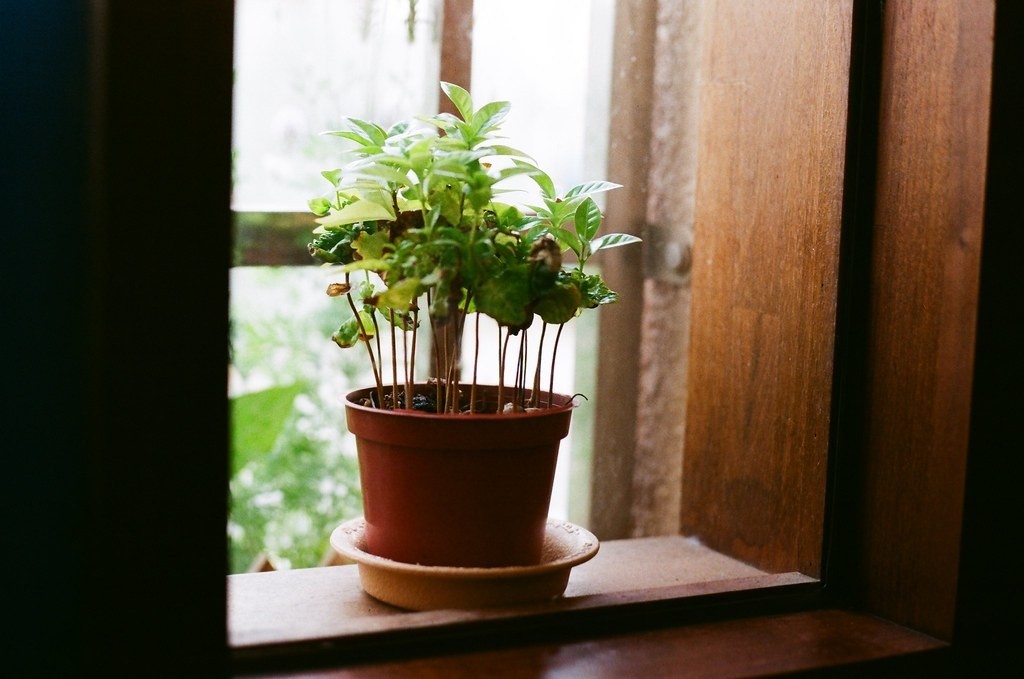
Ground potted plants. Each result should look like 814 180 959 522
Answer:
305 80 644 611
